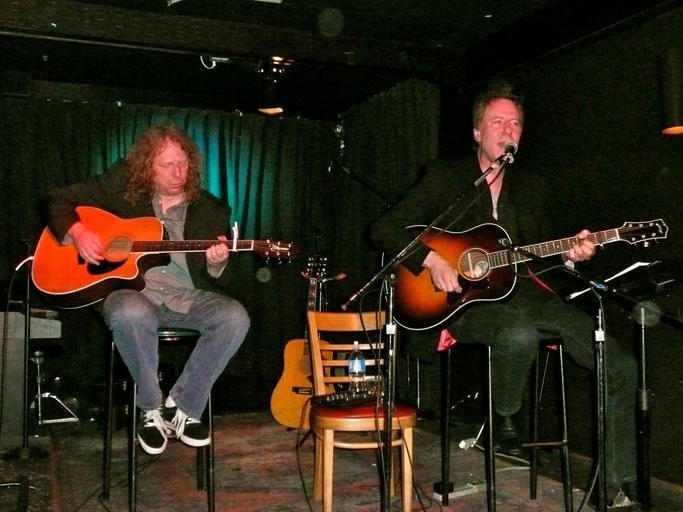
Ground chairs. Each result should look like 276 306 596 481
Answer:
303 308 417 511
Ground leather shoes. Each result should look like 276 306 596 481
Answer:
491 407 524 456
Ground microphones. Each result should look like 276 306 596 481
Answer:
503 138 520 165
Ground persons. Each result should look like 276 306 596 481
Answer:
37 123 253 460
371 81 639 510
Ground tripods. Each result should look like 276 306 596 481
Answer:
31 350 84 438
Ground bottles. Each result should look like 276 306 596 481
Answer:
347 341 366 393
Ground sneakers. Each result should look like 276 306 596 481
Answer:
136 400 177 456
159 399 213 448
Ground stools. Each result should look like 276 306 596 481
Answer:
98 324 218 511
438 326 572 511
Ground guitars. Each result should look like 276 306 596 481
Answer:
270 257 346 429
382 219 669 332
31 205 299 310
